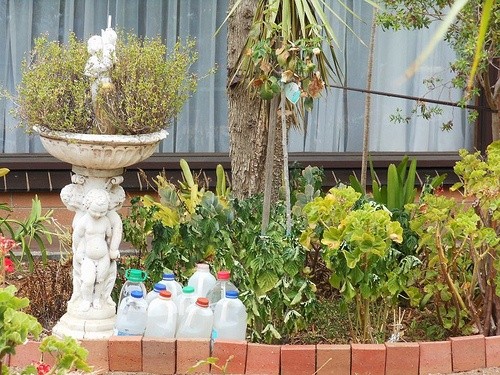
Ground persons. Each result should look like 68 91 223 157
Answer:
85 14 118 112
59 184 128 313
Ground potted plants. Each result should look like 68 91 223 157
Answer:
0 25 221 338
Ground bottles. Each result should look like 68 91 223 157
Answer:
211 290 248 351
118 269 148 310
144 290 178 338
145 283 166 306
157 273 182 312
177 285 197 324
176 297 214 338
205 271 239 314
188 264 217 301
115 291 147 337
